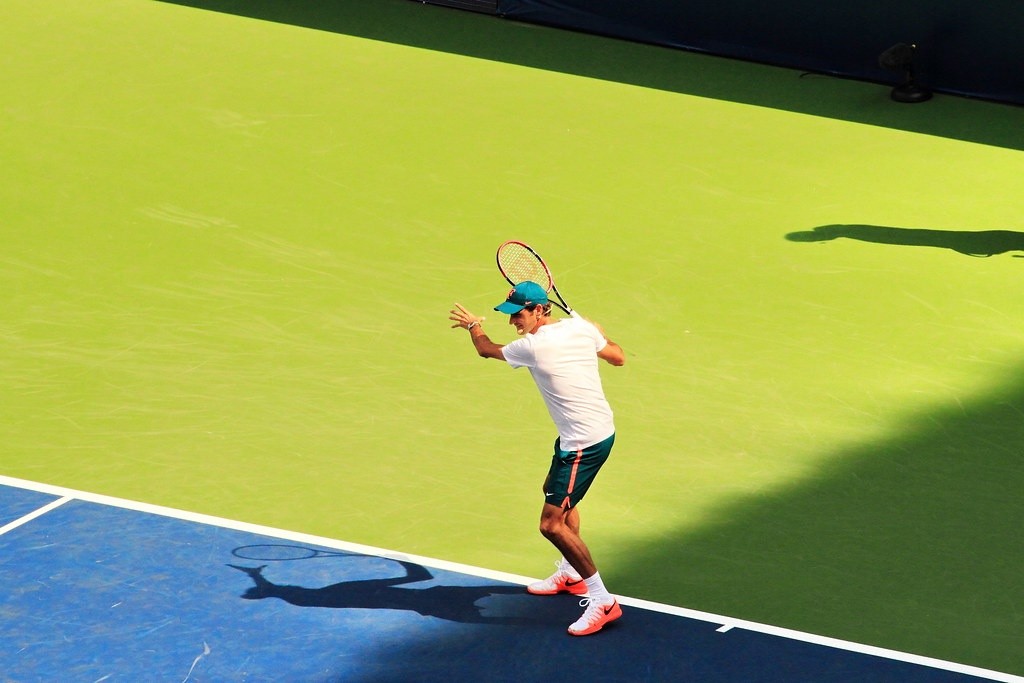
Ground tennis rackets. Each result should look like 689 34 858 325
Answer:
496 239 580 318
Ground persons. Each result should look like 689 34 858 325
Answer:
450 280 625 636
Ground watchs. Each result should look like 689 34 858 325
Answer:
467 321 482 331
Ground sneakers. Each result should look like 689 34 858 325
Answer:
527 559 588 595
567 597 624 636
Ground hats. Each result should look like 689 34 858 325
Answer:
493 281 549 314
